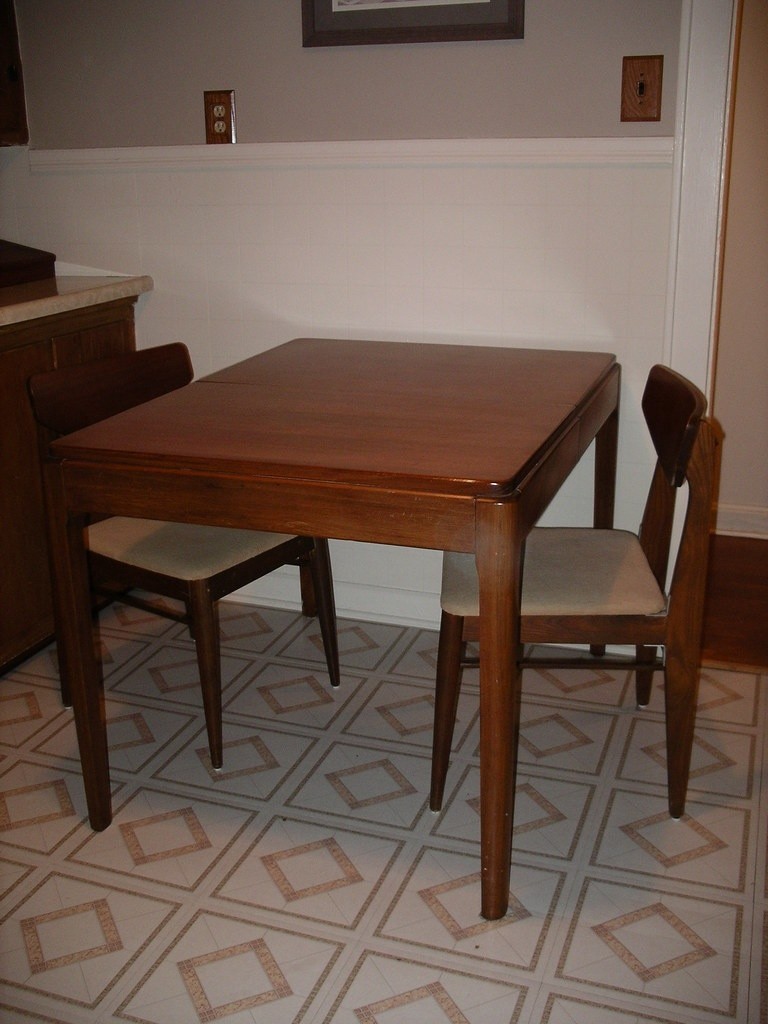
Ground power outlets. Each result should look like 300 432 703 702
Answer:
204 89 236 146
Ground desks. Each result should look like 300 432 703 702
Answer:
43 337 622 922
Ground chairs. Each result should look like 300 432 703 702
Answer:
429 364 726 817
29 341 341 766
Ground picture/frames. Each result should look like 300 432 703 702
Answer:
300 0 525 48
